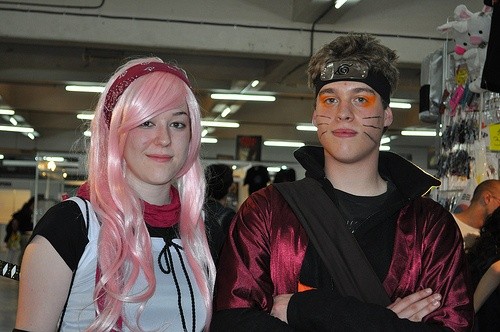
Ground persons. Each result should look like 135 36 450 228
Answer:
198 164 236 265
451 179 500 253
463 206 500 332
215 34 474 331
13 56 216 332
6 194 48 249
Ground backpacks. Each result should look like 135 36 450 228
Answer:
205 210 233 267
